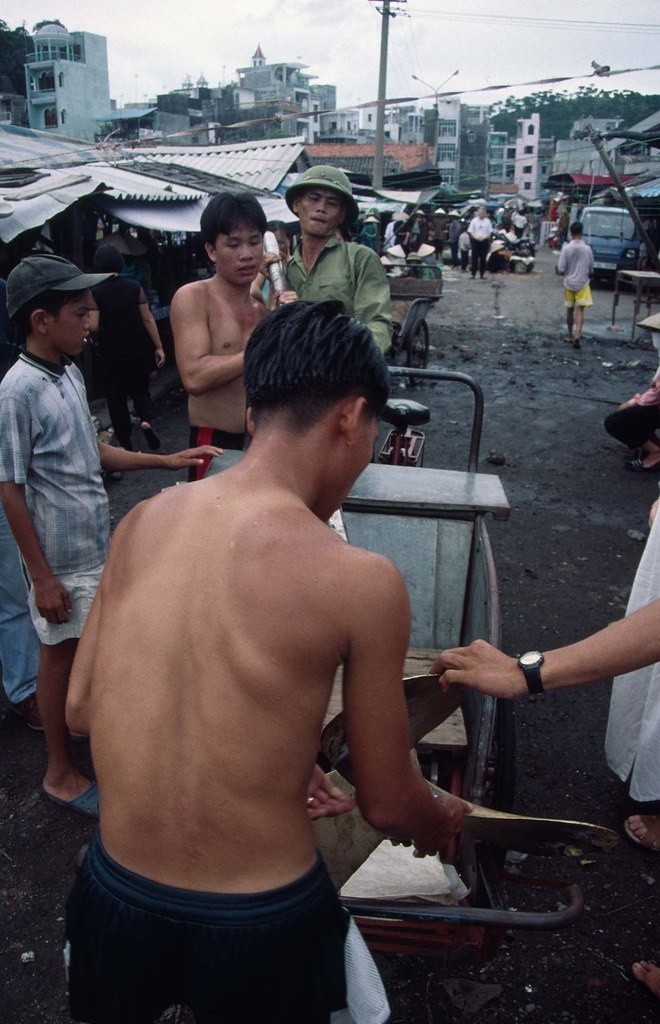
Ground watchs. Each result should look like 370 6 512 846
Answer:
517 650 544 694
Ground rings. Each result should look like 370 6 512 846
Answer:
306 797 314 803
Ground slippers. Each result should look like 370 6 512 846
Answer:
46 779 99 818
625 458 659 473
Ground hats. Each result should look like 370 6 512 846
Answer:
392 212 410 220
406 252 421 261
637 311 659 330
380 255 390 264
418 243 435 257
448 210 460 217
284 164 360 228
386 244 406 258
364 216 379 223
96 232 146 257
415 210 424 216
435 208 445 214
5 253 114 317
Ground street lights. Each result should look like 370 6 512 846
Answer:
411 69 459 167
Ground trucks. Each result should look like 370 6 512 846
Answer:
578 206 642 294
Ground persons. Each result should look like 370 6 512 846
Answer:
169 193 297 483
88 244 165 453
430 485 660 998
556 222 594 350
65 302 474 1024
349 204 569 280
0 254 223 819
0 503 44 731
604 311 660 471
637 217 660 271
259 164 393 357
250 220 294 308
591 192 613 206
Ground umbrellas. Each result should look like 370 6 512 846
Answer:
504 197 528 205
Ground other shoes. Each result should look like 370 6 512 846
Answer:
564 337 573 343
11 698 44 730
143 427 160 450
573 339 580 349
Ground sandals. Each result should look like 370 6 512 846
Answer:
624 814 660 849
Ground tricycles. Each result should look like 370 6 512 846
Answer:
382 264 444 384
202 364 624 1023
